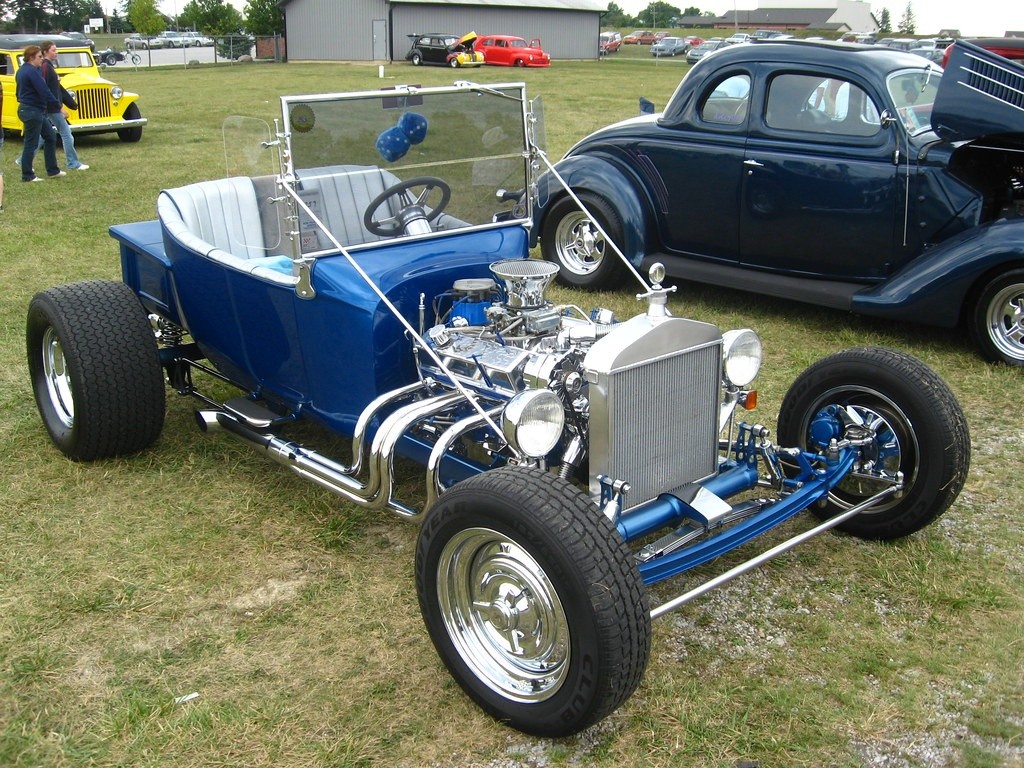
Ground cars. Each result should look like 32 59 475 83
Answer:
403 29 487 68
685 35 705 46
25 81 975 743
123 27 256 51
649 37 690 57
655 31 671 43
491 36 1024 374
623 30 656 46
473 32 551 68
684 40 732 65
710 31 1024 146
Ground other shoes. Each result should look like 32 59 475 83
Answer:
0 205 4 212
74 164 89 170
30 177 44 182
15 160 35 172
49 170 66 178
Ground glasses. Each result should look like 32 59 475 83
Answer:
39 55 42 59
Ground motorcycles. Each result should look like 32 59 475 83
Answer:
92 43 142 65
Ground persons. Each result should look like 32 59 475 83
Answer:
0 82 4 212
13 40 89 181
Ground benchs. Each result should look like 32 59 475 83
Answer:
154 163 394 261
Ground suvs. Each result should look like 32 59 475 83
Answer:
601 31 623 55
59 30 96 52
0 32 148 147
